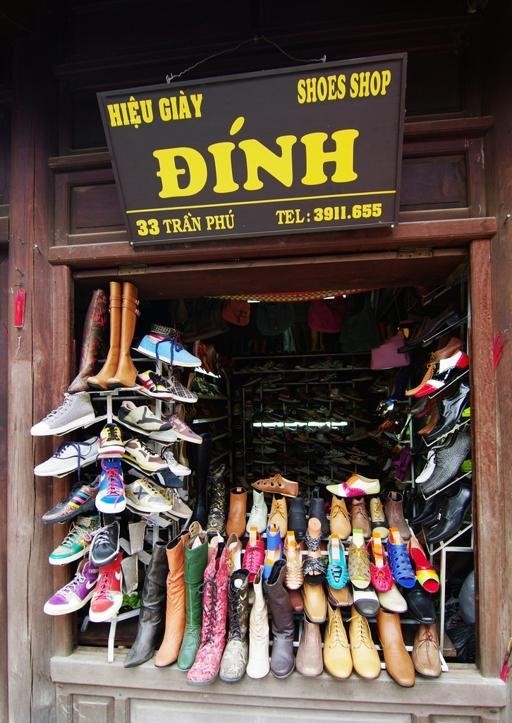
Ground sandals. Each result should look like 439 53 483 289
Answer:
348 531 373 592
300 528 329 586
405 533 444 594
384 528 418 591
321 532 351 590
223 528 244 573
281 532 303 590
366 534 395 592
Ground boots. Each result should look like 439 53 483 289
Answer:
123 538 169 672
65 284 110 396
87 277 121 391
186 538 231 686
294 608 324 680
106 278 142 391
263 558 298 680
217 566 252 684
176 532 209 673
245 564 273 680
153 529 190 671
376 598 418 689
321 602 354 681
346 599 383 682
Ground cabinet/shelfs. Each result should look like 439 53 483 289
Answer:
393 287 480 654
78 361 235 664
229 351 367 503
203 510 450 676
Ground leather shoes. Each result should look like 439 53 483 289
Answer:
260 524 286 582
350 584 382 618
301 580 328 624
324 582 355 609
286 586 307 613
242 530 266 583
374 582 410 615
410 618 445 681
405 581 439 626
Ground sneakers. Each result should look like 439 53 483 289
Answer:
94 456 128 515
37 474 104 526
39 559 102 618
96 418 128 460
33 435 102 478
26 391 99 437
46 513 104 569
111 276 473 547
87 552 124 625
88 515 122 566
